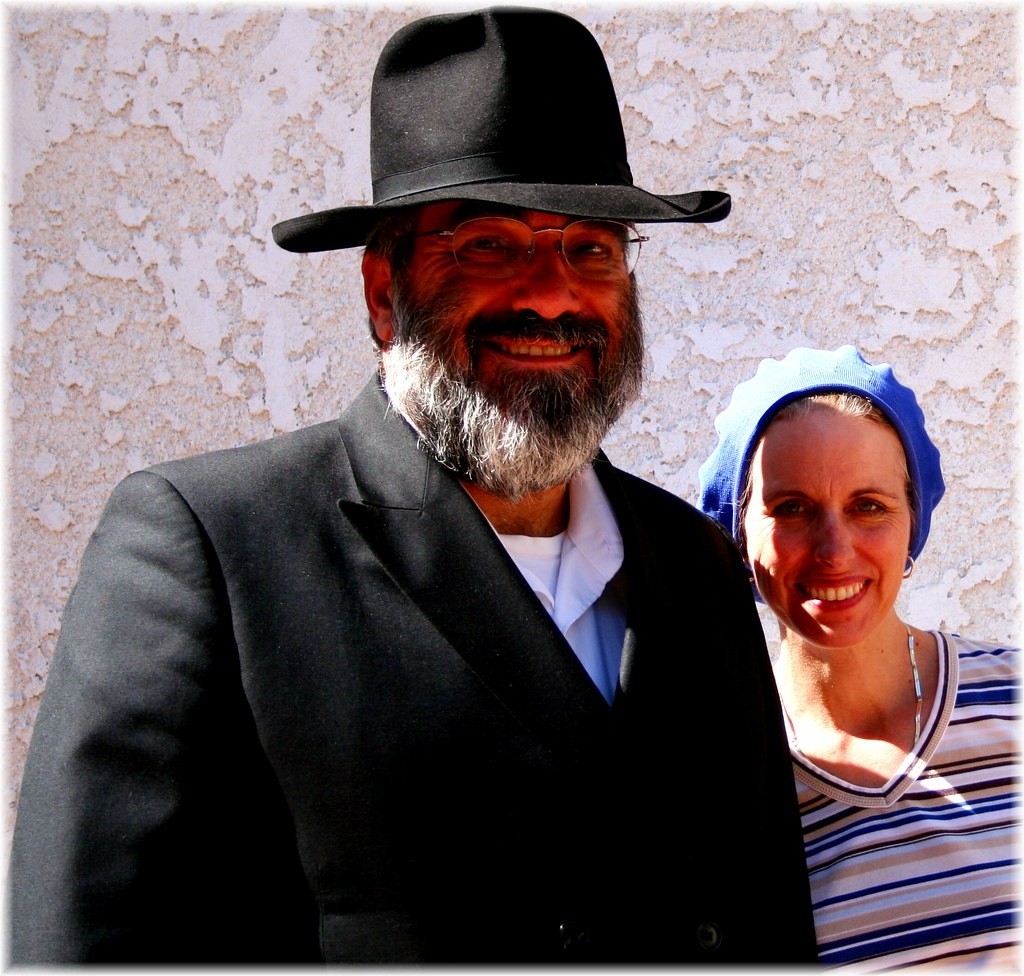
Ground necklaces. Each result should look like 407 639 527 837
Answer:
778 624 923 769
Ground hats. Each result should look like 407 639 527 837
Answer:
698 345 945 603
272 8 732 251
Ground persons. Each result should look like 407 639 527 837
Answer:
6 8 822 969
698 346 1017 965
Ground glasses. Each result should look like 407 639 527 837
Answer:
400 218 648 279
